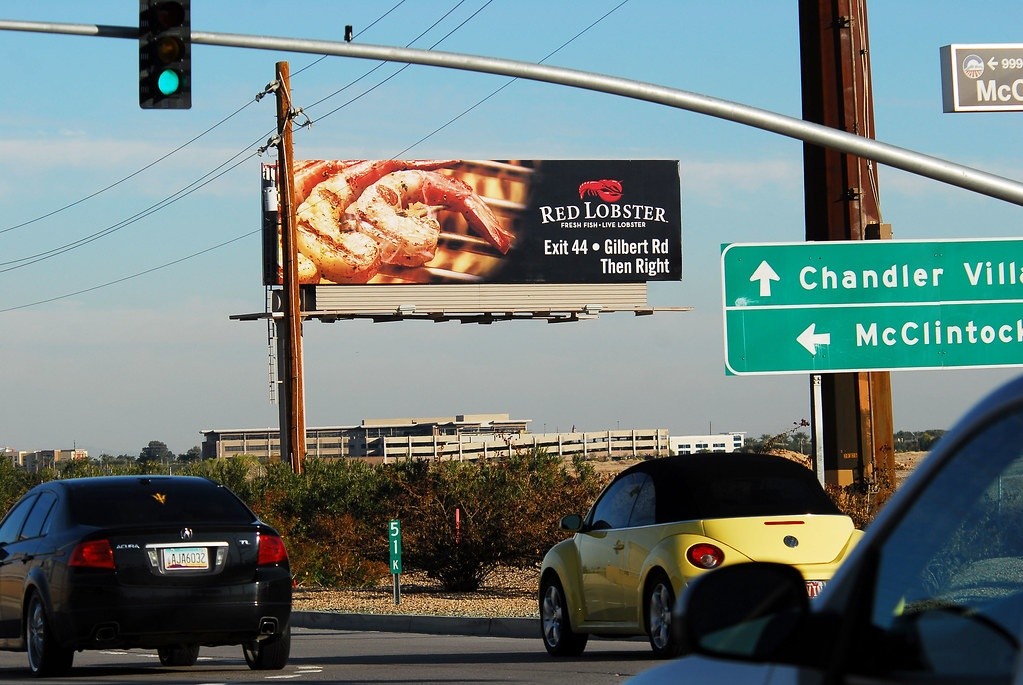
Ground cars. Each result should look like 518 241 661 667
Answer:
615 373 1023 684
538 451 905 656
0 475 294 673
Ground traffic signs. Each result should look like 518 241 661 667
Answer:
722 239 1023 375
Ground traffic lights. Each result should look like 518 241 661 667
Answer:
138 0 192 107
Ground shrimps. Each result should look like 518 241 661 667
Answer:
291 159 517 284
